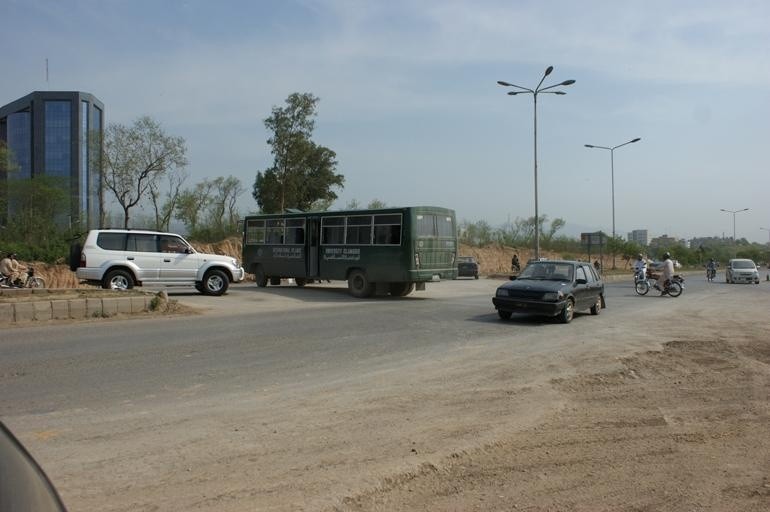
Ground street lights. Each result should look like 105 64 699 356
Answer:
721 208 748 258
585 138 642 269
498 65 576 260
760 228 770 242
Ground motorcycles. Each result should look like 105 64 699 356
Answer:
707 266 717 281
630 263 647 285
0 264 46 289
635 268 685 297
511 264 519 272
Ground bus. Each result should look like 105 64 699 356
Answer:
236 205 459 298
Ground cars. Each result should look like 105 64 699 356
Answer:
458 256 480 279
492 258 606 324
726 258 761 284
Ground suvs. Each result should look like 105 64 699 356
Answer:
69 229 245 296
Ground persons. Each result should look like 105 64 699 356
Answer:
511 254 521 272
161 239 171 253
707 258 717 278
630 252 651 289
11 253 27 282
593 260 601 272
0 253 20 286
653 252 674 297
532 264 547 276
